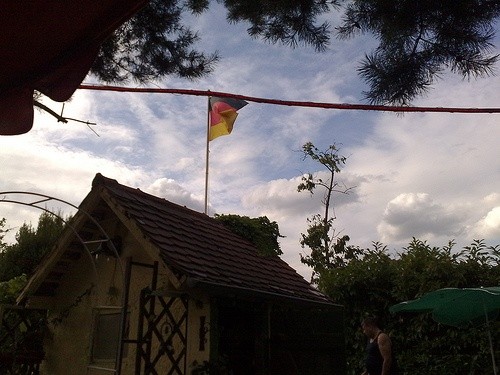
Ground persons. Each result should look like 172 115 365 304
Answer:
359 317 392 375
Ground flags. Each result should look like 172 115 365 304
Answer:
209 95 249 142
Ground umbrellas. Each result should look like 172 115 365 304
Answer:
390 285 500 375
